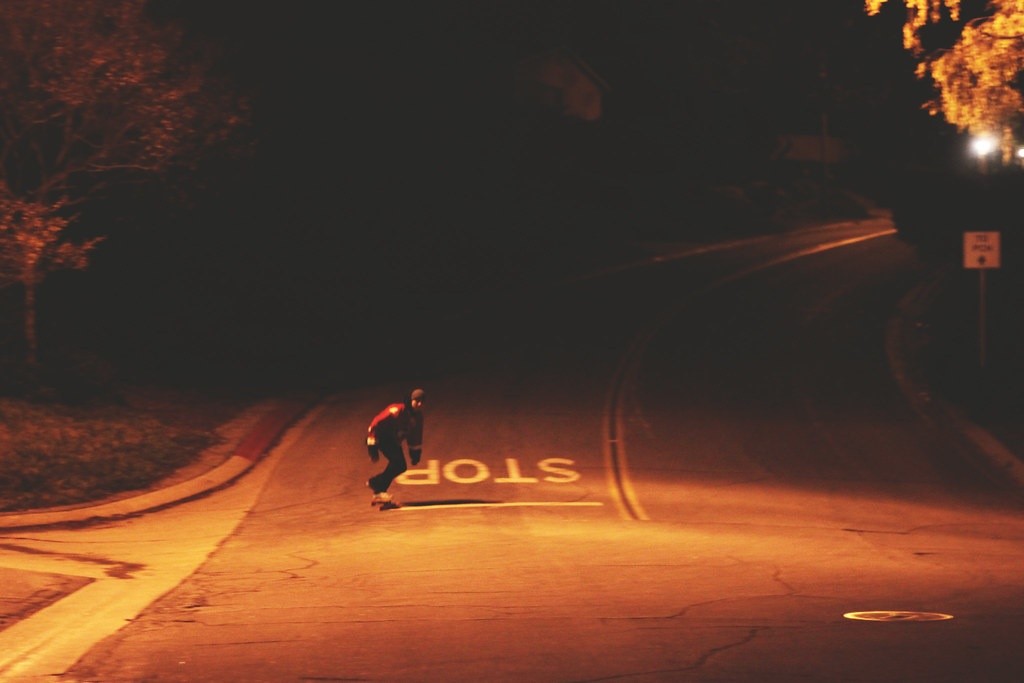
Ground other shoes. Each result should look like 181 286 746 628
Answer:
371 493 392 503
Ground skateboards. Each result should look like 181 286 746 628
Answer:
370 494 403 513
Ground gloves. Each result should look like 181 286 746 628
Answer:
409 449 421 465
368 445 379 462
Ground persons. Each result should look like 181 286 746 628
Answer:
365 389 426 500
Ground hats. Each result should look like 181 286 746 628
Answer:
411 389 426 400
